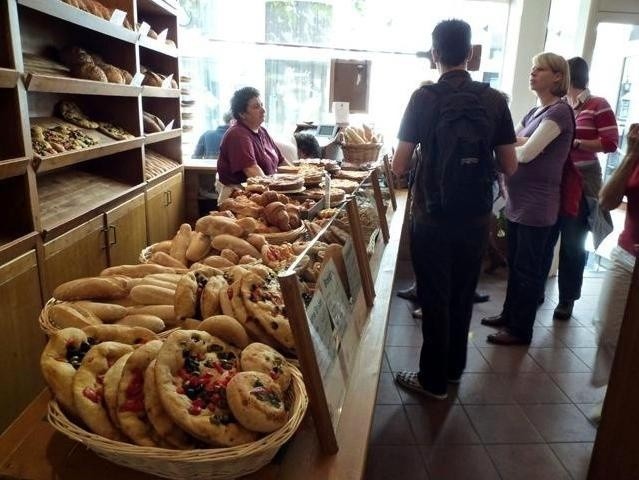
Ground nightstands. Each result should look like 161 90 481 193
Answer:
0 1 185 428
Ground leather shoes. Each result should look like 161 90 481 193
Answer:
481 312 508 326
412 306 422 317
398 282 417 299
488 328 531 345
472 291 489 302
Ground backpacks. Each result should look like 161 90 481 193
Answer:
409 80 495 225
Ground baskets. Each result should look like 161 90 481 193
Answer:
139 240 269 270
260 219 308 246
287 359 301 368
341 141 383 164
38 297 183 339
46 358 308 480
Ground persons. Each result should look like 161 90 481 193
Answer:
295 132 322 160
480 52 578 347
586 124 637 428
190 110 236 161
537 57 621 322
214 85 296 206
391 21 518 400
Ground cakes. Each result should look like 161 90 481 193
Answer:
318 178 359 193
247 172 305 191
335 170 372 183
277 157 342 184
302 187 346 209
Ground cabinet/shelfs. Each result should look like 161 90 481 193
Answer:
184 157 219 235
1 150 409 479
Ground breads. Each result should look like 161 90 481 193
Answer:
145 147 180 180
210 183 343 232
165 39 177 50
344 123 383 144
196 315 249 351
226 342 291 432
173 265 314 359
64 0 133 30
129 63 178 89
146 215 351 283
40 324 257 450
73 47 133 85
49 263 184 332
143 110 166 132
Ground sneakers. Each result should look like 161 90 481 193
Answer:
397 371 449 400
449 375 462 383
554 299 573 318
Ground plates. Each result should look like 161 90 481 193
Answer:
242 183 305 193
